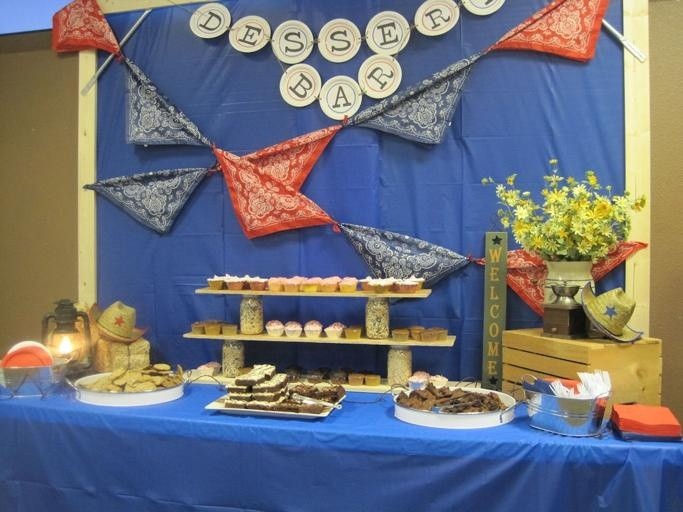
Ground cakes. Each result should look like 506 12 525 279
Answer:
264 319 364 339
285 367 381 386
225 364 345 416
408 370 448 390
191 320 238 336
196 361 222 376
206 273 426 294
391 325 449 343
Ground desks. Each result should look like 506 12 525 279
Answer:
1 368 683 512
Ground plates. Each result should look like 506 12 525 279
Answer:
3 341 54 366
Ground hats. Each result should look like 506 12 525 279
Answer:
89 301 148 343
581 282 643 342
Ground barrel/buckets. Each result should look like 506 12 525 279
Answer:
520 373 612 437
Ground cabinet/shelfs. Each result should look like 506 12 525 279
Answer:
501 327 663 419
181 288 481 395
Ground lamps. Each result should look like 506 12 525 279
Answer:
41 299 92 368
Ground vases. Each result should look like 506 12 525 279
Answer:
543 261 596 304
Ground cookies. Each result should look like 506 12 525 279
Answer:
76 363 184 394
395 382 507 415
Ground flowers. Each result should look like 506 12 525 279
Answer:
481 159 646 264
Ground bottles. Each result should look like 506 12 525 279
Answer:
386 346 413 387
240 294 265 335
221 340 245 379
365 298 390 339
